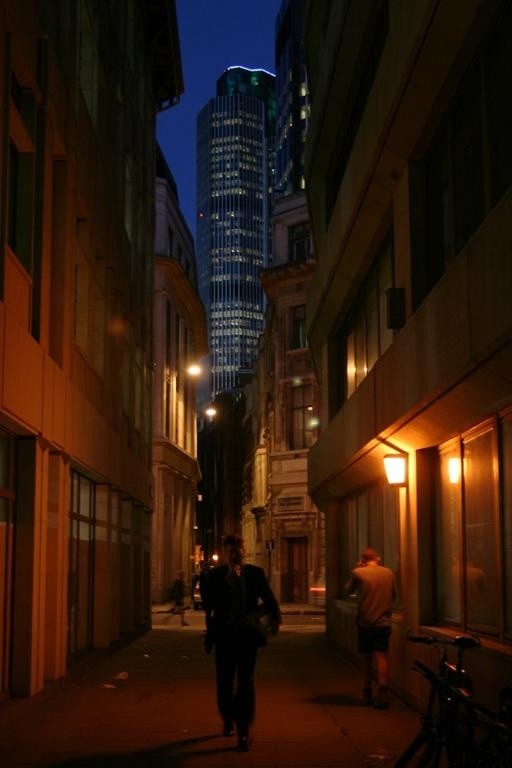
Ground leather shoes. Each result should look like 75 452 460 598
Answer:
221 721 249 752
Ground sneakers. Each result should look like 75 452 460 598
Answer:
360 688 390 707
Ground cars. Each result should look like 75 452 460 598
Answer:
193 580 205 608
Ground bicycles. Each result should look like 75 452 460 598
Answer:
395 631 511 767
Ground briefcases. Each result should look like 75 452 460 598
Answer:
250 603 281 645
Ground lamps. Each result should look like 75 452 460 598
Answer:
382 453 408 488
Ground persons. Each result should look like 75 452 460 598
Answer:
158 569 191 627
201 532 282 753
339 547 400 712
